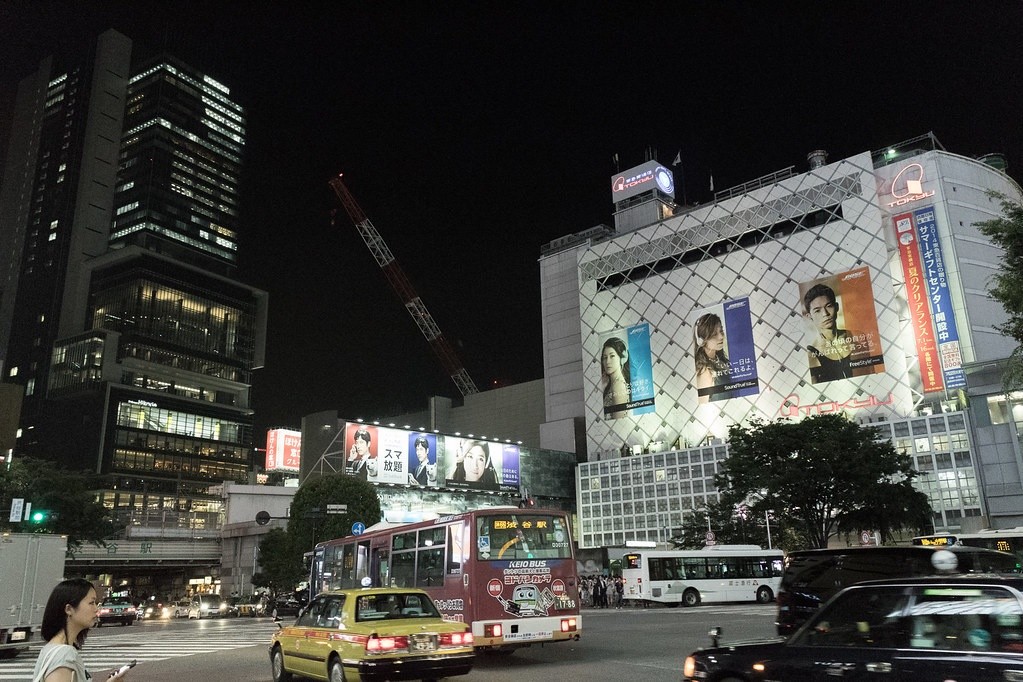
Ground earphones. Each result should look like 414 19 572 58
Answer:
808 315 811 320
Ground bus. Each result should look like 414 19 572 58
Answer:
910 526 1023 563
302 505 582 658
621 544 787 608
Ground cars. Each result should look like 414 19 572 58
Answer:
93 596 136 628
267 586 478 682
135 581 310 622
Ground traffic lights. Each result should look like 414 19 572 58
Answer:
30 511 59 523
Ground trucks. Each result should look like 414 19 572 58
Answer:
0 530 70 658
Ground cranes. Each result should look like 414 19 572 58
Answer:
329 177 480 397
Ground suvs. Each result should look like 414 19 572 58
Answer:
680 584 1023 682
774 544 1023 642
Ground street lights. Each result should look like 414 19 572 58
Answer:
765 510 775 576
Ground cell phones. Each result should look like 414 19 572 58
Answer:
108 660 137 680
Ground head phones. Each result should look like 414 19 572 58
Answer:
620 339 629 365
695 319 706 348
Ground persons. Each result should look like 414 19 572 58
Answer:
600 337 631 420
411 437 432 486
577 574 624 609
346 429 372 481
230 591 239 597
448 438 499 485
693 313 733 403
32 578 125 682
804 284 875 385
